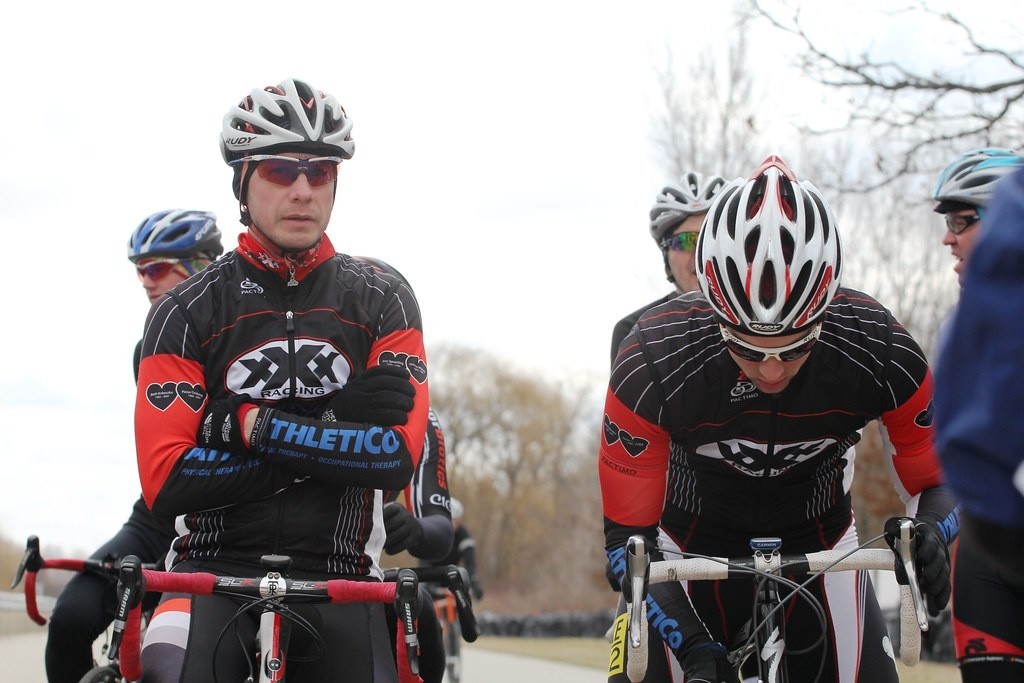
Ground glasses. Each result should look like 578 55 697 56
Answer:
232 154 343 187
659 229 699 253
137 257 199 283
942 212 983 236
718 322 822 362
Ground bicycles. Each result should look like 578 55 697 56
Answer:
618 518 931 682
8 533 481 683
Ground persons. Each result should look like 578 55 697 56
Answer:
609 170 729 381
441 497 483 683
599 151 958 683
379 404 456 683
933 148 1024 288
134 79 427 683
933 154 1024 600
44 200 225 683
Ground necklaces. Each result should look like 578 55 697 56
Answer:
246 230 325 287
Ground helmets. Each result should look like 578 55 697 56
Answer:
450 497 462 519
649 172 730 240
932 149 1024 217
128 207 224 261
219 79 355 162
695 154 842 332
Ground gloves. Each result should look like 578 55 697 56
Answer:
196 390 255 455
377 501 421 556
682 646 738 683
318 363 415 426
884 515 951 617
